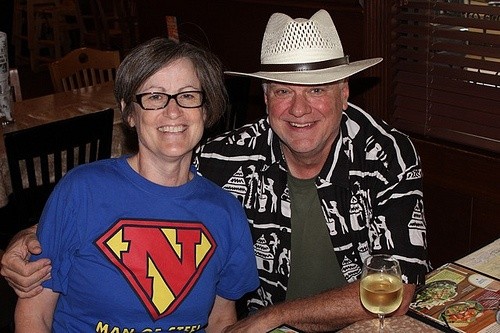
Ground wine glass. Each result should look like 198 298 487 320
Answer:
359 254 403 333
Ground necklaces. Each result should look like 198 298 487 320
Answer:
279 144 287 162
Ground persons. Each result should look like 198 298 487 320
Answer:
1 7 429 332
13 36 262 333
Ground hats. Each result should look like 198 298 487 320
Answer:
223 9 383 86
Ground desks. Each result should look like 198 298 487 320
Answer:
0 80 124 154
336 238 500 333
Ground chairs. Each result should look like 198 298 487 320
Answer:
48 47 121 93
9 68 23 103
3 108 114 235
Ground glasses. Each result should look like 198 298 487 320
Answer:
133 86 207 110
